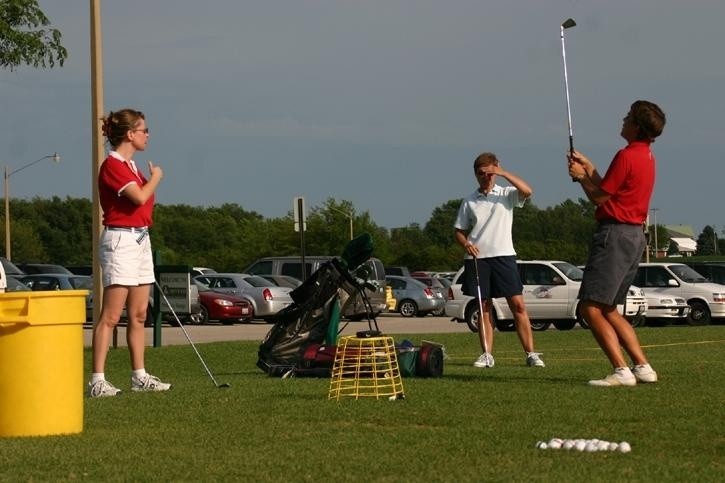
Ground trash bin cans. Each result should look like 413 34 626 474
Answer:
0 290 90 437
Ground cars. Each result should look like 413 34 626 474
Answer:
680 262 725 287
188 278 252 324
4 274 31 294
193 271 294 323
19 273 128 319
384 266 410 277
384 273 445 317
527 274 693 330
257 274 303 293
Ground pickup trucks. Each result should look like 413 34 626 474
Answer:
124 282 200 327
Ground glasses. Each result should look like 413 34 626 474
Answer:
133 128 148 132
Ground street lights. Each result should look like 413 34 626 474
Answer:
320 199 354 246
4 150 61 261
650 206 661 257
713 222 721 255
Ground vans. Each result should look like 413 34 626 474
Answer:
411 270 440 280
0 255 24 280
15 264 74 276
432 271 458 278
240 255 387 321
192 266 218 278
567 263 725 325
64 265 94 275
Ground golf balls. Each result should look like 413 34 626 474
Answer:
536 438 632 454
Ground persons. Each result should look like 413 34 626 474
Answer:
565 98 668 386
89 107 173 396
453 151 546 367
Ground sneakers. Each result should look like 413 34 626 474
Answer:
473 352 495 368
131 374 173 392
88 380 123 398
589 365 657 386
526 351 545 367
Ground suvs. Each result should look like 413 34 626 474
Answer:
412 277 453 316
448 258 648 331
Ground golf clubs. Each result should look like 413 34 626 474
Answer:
353 265 379 292
473 257 493 368
155 280 230 387
561 17 577 183
